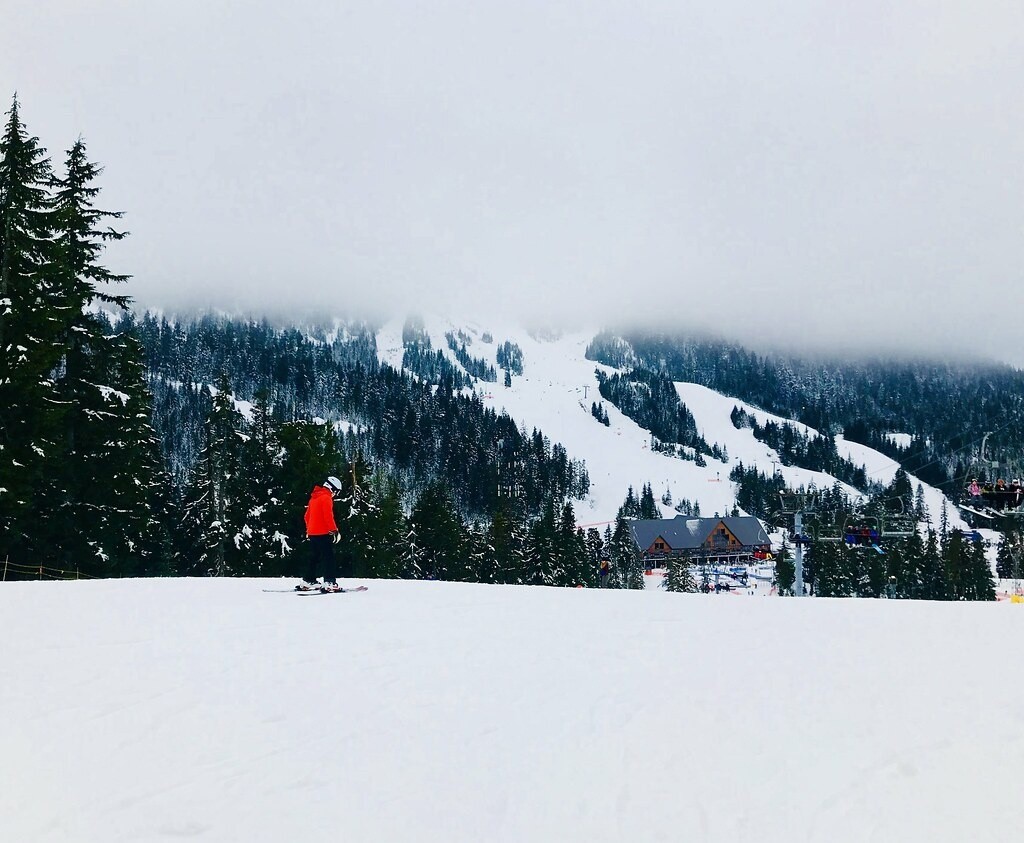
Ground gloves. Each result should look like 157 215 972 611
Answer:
306 531 311 541
329 529 341 544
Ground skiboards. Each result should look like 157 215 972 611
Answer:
262 583 368 596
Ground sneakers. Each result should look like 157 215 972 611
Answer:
316 577 338 593
300 577 322 590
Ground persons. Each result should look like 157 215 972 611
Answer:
304 476 343 594
703 559 770 596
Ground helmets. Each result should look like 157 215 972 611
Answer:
323 476 342 495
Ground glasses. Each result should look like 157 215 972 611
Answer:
336 489 340 496
